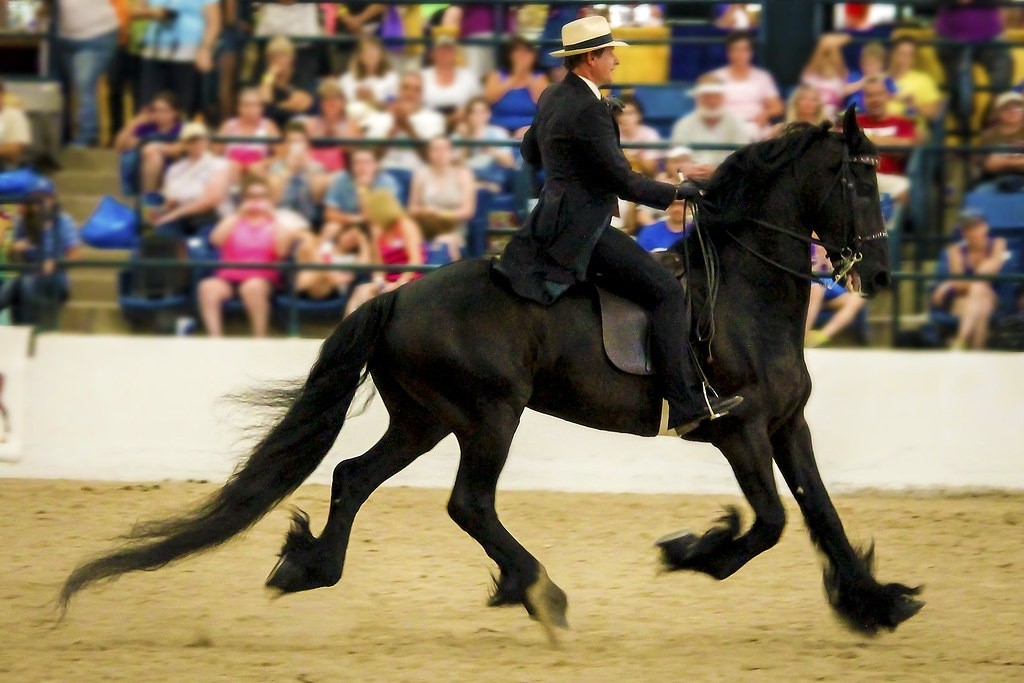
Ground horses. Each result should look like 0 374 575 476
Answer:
50 100 928 639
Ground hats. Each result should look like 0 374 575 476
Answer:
548 16 630 57
683 72 734 97
993 90 1024 109
180 120 206 141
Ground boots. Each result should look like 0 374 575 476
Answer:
647 319 744 436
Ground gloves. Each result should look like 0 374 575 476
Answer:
675 180 700 200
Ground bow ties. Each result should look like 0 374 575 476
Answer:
601 95 625 115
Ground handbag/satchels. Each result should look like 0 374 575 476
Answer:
79 196 143 247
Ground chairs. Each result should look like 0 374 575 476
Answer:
0 0 1024 350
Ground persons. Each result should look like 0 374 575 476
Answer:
0 0 1024 352
493 15 744 435
